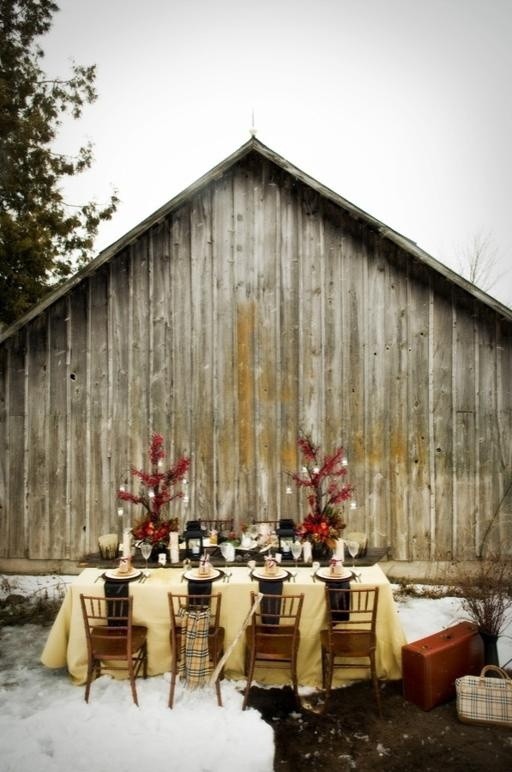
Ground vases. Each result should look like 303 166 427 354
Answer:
479 631 500 677
146 543 164 563
313 545 334 561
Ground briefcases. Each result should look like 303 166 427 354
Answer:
401 621 486 712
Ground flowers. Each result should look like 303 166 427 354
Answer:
283 429 355 545
118 432 190 542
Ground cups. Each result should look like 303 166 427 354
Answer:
248 525 261 540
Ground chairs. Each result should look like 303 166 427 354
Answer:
320 588 380 708
81 593 149 706
167 591 225 709
240 592 306 711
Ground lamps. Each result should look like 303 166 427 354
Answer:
187 520 204 558
278 518 296 556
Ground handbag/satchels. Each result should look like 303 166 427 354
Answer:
454 664 512 727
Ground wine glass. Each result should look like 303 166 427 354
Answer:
346 540 361 571
140 545 154 577
219 542 233 573
291 542 302 575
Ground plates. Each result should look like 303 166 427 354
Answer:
315 567 353 581
103 568 140 581
181 567 220 582
250 566 288 580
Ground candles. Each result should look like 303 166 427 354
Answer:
169 530 179 563
303 540 312 563
334 539 344 562
121 530 131 561
